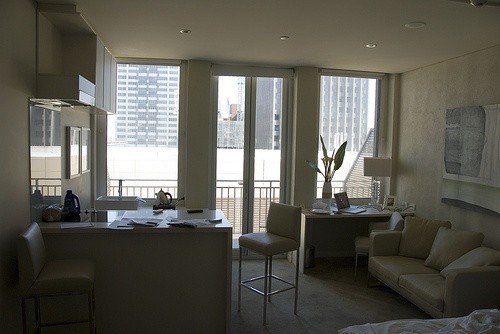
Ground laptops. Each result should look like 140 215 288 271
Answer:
334 192 366 214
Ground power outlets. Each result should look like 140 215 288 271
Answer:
401 201 408 208
408 203 416 211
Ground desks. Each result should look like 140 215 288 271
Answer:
337 311 500 334
95 195 139 210
291 204 415 274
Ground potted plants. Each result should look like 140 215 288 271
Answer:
305 134 347 206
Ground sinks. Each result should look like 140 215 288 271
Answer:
95 195 139 210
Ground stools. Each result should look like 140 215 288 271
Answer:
237 202 302 328
17 222 98 334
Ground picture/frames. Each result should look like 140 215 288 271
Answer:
382 195 398 209
333 192 351 210
81 126 91 174
66 125 82 179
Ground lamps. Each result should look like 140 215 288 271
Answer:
363 156 391 209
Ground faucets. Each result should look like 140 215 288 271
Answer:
117 179 123 201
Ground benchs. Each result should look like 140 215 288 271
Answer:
366 197 500 319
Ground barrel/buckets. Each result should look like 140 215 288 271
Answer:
62 190 80 222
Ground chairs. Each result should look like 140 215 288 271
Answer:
354 211 404 287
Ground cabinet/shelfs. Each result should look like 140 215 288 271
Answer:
34 2 118 116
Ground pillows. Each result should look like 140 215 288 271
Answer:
423 227 485 271
439 246 500 278
398 216 451 260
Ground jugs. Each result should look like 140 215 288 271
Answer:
154 188 172 205
